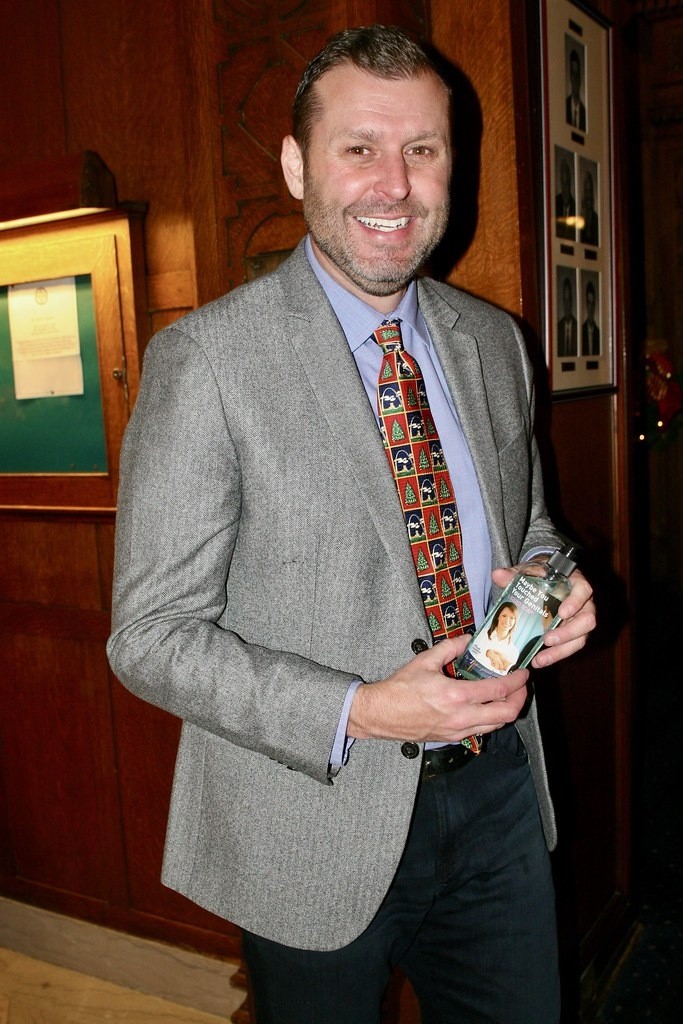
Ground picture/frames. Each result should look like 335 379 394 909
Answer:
532 0 618 404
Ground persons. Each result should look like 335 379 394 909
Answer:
474 601 519 677
582 282 599 355
106 23 595 1024
507 592 562 676
556 159 576 240
558 277 577 357
580 171 598 246
566 49 586 131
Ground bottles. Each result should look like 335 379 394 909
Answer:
456 543 580 688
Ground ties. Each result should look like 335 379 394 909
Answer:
369 319 484 761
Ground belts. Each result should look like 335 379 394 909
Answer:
419 737 487 784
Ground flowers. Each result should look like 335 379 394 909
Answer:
633 335 683 455
